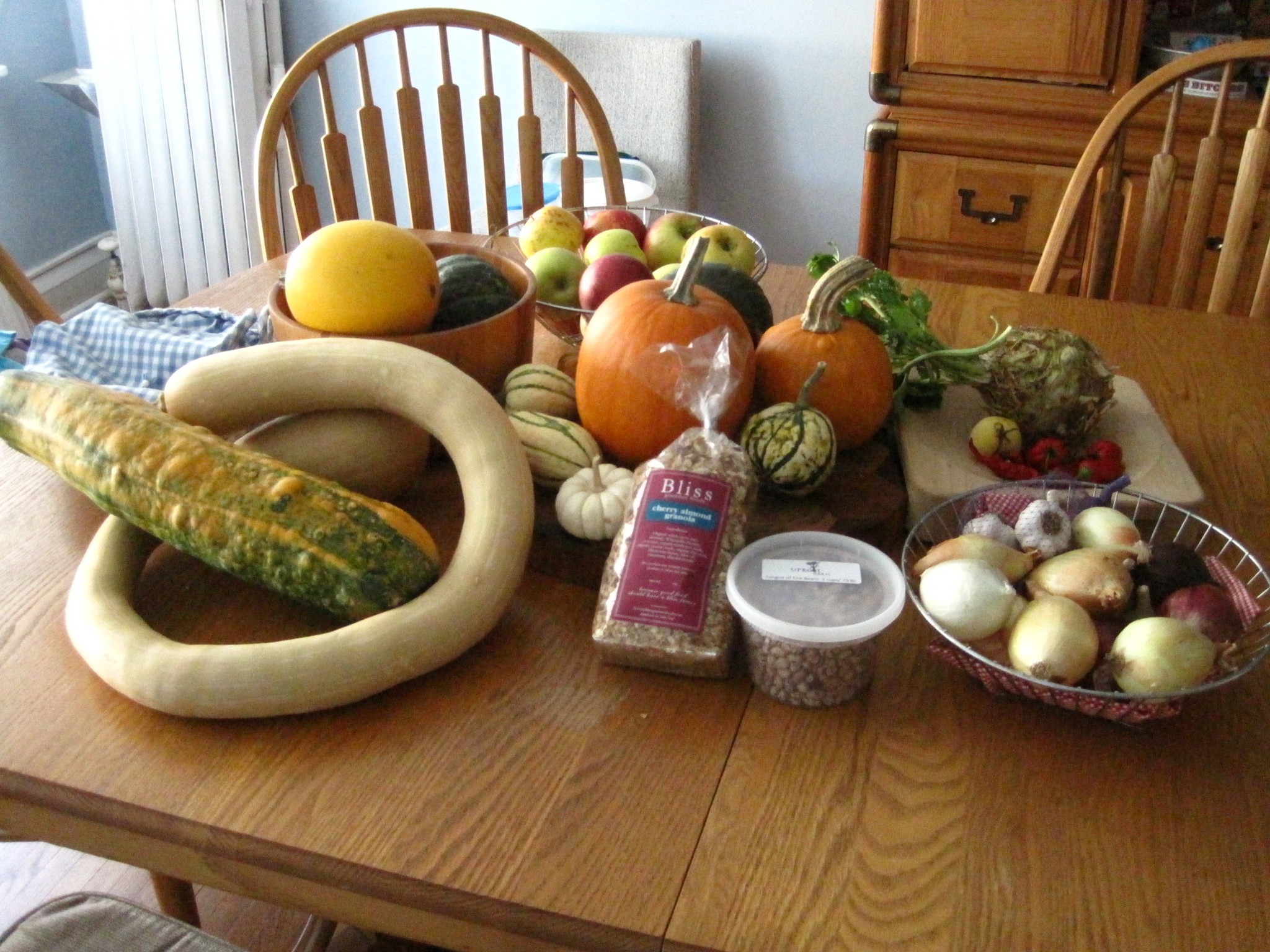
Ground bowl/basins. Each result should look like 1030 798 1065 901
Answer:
269 244 535 407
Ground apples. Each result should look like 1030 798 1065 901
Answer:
517 196 749 319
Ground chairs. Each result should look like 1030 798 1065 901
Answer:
1026 40 1270 321
247 4 634 262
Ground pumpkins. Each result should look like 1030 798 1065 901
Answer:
636 261 771 364
499 407 603 481
735 359 841 496
556 452 637 546
502 353 574 420
571 233 751 471
751 258 903 460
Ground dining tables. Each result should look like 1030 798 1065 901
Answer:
1 222 1270 952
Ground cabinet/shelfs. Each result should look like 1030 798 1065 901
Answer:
852 1 1267 323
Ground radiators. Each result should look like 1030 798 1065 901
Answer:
81 0 290 316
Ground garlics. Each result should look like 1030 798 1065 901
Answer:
1015 497 1076 558
965 514 1016 548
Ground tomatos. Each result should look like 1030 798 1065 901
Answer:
964 413 1127 488
